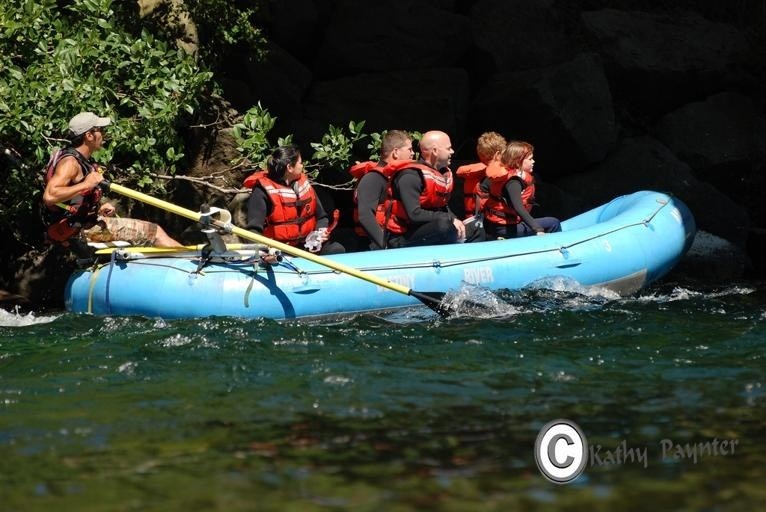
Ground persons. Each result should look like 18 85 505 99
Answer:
340 128 417 252
243 145 346 264
479 139 563 239
375 127 485 250
39 110 184 259
463 129 508 241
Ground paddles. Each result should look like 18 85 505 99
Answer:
94 243 269 254
99 180 494 319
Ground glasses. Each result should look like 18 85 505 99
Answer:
90 126 103 132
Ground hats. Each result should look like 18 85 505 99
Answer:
68 112 111 138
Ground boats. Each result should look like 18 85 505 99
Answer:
64 190 696 320
